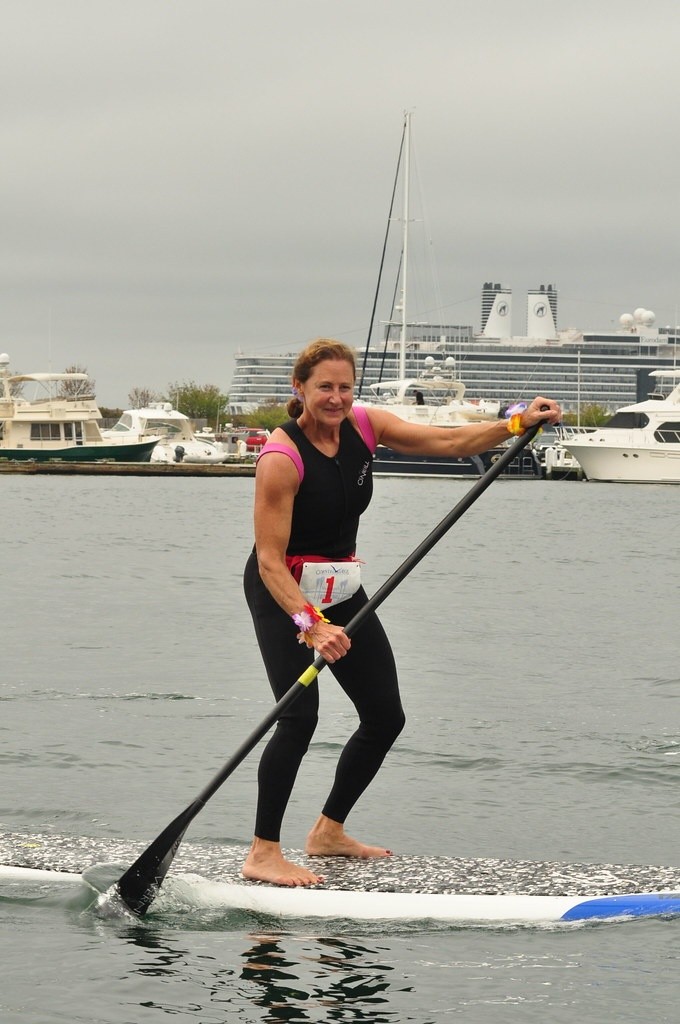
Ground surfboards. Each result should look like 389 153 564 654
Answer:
0 831 680 919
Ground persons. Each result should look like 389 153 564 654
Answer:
244 339 563 891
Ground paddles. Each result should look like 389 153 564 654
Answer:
117 404 562 918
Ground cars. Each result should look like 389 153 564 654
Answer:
232 428 268 452
536 446 561 461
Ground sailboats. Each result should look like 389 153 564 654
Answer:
348 115 542 484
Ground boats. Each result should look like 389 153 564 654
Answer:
107 401 229 463
564 369 680 485
1 354 161 462
